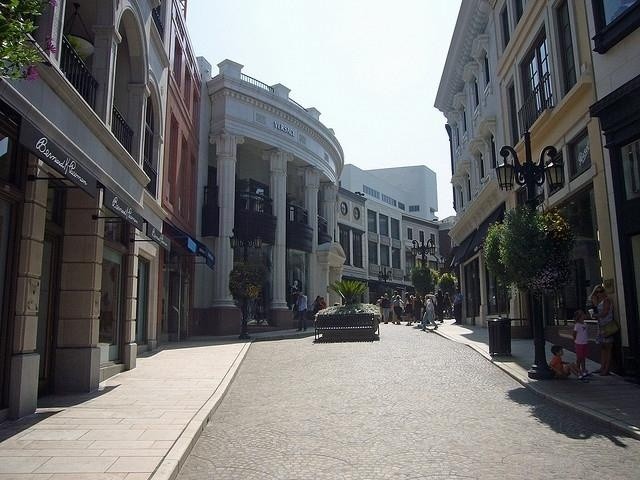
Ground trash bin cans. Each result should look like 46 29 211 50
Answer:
486 316 512 356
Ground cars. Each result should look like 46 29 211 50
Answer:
443 306 454 319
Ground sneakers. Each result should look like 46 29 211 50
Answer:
576 369 609 379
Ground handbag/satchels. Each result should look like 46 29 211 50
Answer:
604 321 618 336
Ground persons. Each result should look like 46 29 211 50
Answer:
313 296 320 315
374 290 462 330
588 285 614 376
318 297 326 310
549 345 590 380
297 294 307 331
572 309 588 377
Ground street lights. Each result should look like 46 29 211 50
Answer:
494 120 565 380
378 267 392 297
228 213 263 340
410 230 436 324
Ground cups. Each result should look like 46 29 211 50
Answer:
588 308 595 319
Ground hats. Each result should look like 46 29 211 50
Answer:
592 285 605 296
